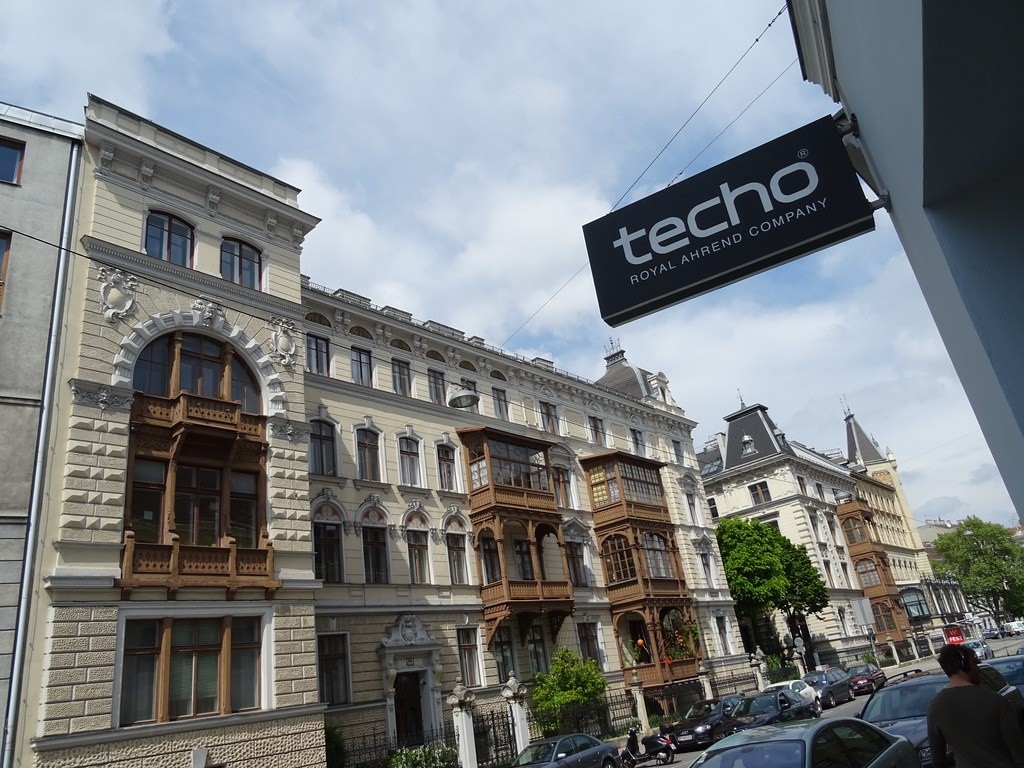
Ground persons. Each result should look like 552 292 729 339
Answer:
999 625 1005 638
926 644 1024 768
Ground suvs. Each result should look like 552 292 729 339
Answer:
801 667 855 708
853 663 1024 768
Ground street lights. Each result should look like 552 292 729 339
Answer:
867 628 881 668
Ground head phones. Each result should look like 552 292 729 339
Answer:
955 646 971 672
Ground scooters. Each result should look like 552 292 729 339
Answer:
621 722 677 768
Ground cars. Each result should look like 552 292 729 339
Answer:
688 717 922 768
982 628 1005 638
981 655 1024 696
761 680 822 713
713 690 820 742
960 640 995 661
1008 621 1024 633
673 694 747 750
509 733 623 768
845 664 888 694
